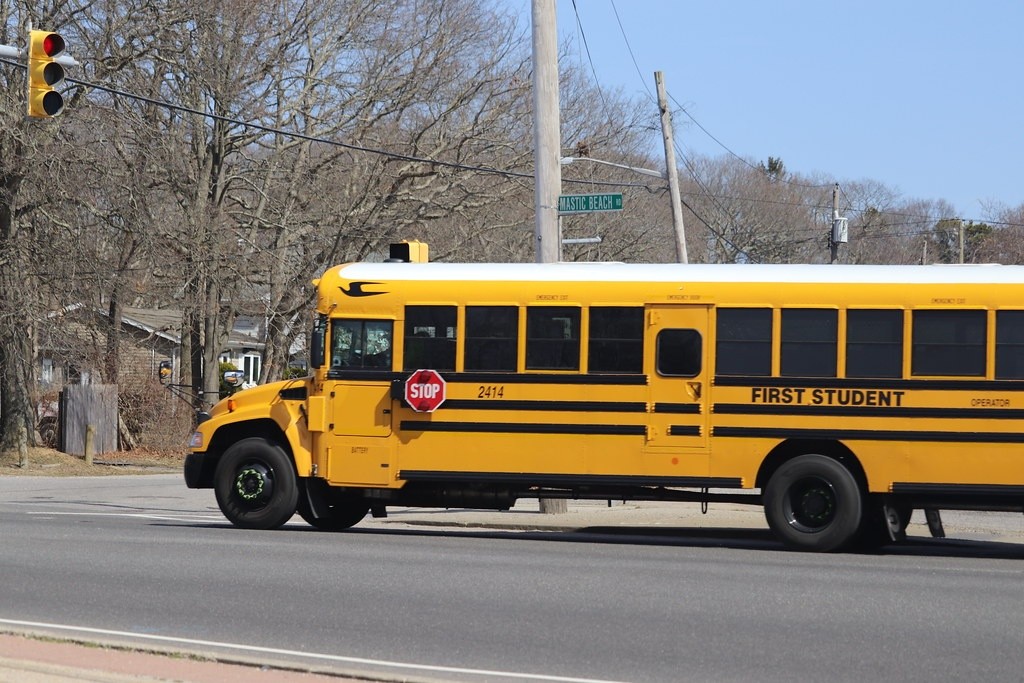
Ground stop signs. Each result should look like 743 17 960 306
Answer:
405 368 447 413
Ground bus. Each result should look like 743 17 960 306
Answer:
156 235 1024 553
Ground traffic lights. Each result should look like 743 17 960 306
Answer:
25 28 67 121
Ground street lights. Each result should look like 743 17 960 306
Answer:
559 154 691 265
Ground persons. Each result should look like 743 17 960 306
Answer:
330 307 1023 384
901 508 946 538
